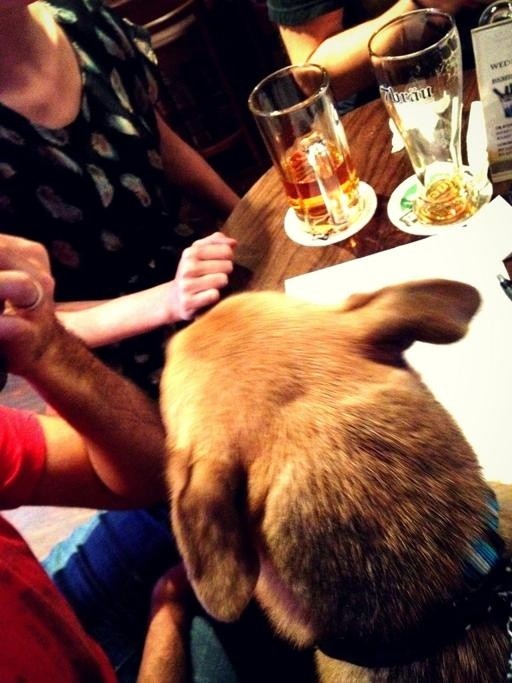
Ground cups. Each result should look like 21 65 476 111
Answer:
248 64 364 235
366 9 478 224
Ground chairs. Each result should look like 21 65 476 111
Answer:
138 2 262 238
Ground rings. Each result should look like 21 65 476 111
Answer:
11 279 44 314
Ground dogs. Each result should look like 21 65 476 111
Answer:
158 278 512 683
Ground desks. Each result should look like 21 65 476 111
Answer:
193 67 512 487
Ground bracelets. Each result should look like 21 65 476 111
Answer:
412 0 436 31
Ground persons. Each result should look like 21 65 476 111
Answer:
266 0 467 120
0 233 278 683
0 2 241 408
